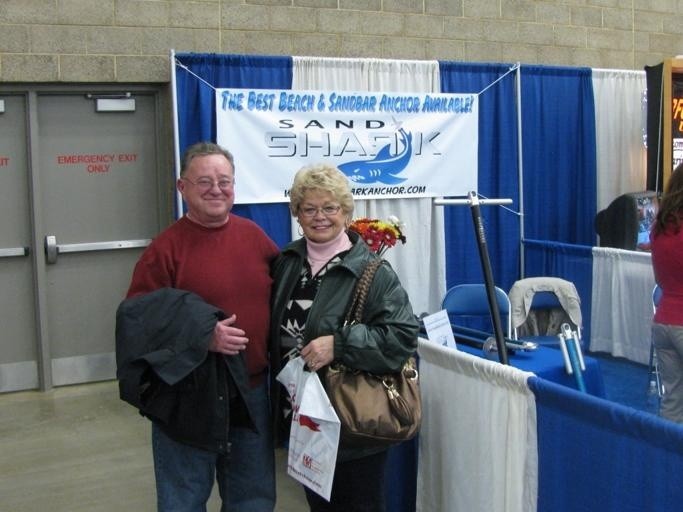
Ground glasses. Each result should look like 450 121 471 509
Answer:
299 205 341 216
185 177 234 187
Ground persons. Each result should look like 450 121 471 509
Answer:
266 164 423 511
648 164 683 423
125 141 280 511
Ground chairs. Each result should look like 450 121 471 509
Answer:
440 276 589 394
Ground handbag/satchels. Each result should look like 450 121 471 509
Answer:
324 258 422 444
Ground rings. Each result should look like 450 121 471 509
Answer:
309 360 315 367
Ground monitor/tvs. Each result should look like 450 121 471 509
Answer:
594 191 659 252
